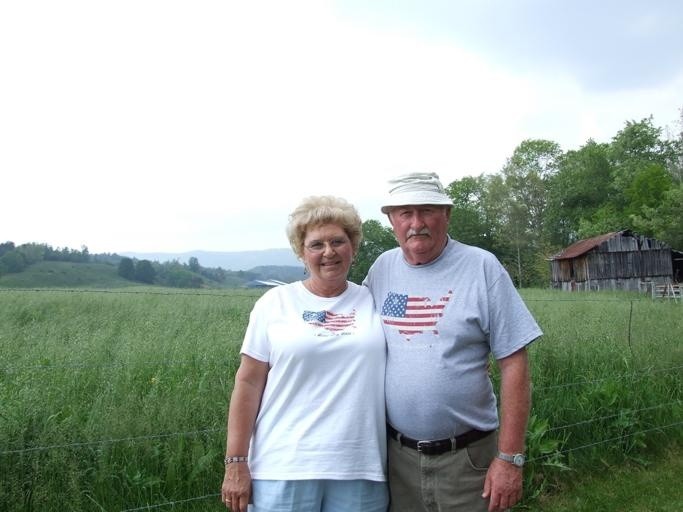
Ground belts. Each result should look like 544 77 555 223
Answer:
387 423 496 455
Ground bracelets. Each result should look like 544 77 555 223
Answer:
222 456 248 463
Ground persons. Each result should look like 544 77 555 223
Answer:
363 170 543 512
219 191 388 512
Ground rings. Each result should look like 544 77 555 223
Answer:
225 499 231 503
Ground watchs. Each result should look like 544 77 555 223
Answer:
497 451 525 467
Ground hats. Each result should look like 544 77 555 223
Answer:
381 172 455 214
